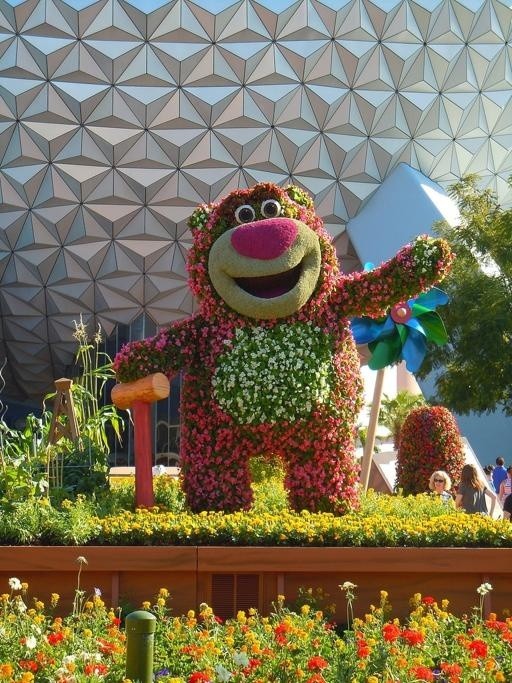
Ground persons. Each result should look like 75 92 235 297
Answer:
430 471 455 504
454 464 496 519
483 456 511 524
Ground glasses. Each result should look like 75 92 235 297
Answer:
433 479 444 482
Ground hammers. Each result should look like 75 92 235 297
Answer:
111 374 171 507
